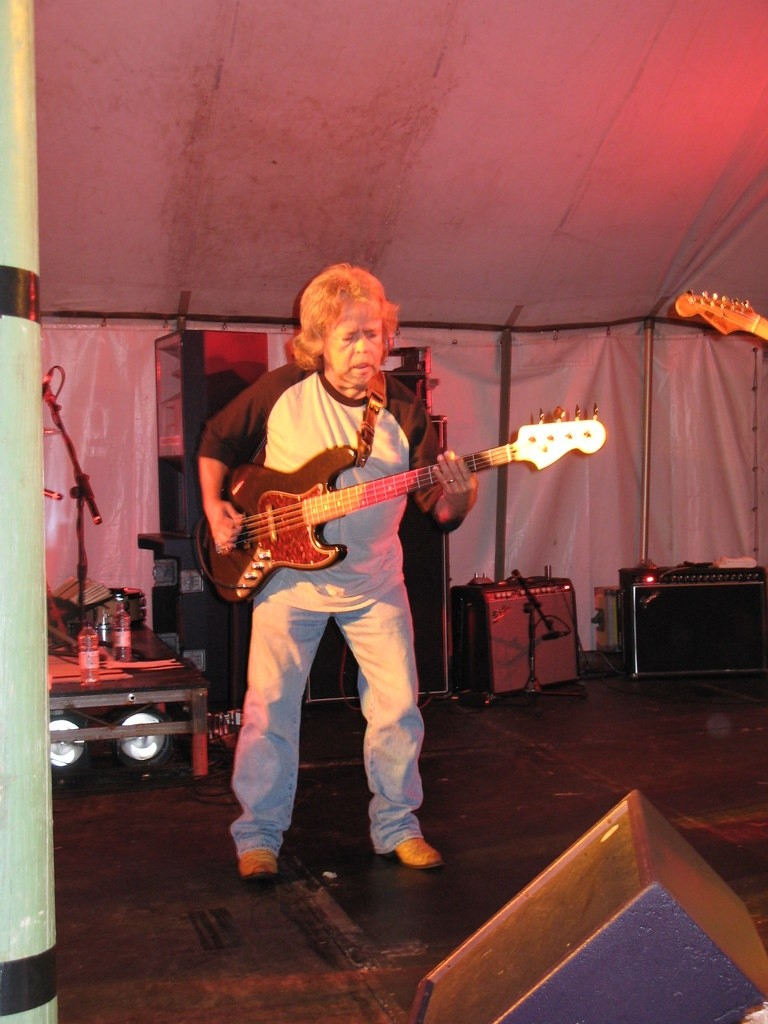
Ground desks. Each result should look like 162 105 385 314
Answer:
47 622 210 778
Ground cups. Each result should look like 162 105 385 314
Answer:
97 623 112 646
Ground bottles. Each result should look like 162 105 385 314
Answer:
112 603 131 661
78 623 99 686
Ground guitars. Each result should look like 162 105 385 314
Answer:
186 403 609 603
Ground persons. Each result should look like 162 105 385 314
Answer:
195 262 479 881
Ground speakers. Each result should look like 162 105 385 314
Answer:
618 565 767 680
450 575 581 696
405 787 767 1024
303 415 452 705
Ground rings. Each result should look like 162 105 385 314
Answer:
447 479 454 483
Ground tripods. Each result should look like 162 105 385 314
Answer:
483 569 586 706
44 386 145 661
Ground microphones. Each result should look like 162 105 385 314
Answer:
42 366 55 398
541 630 568 641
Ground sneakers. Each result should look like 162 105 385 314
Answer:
394 837 444 869
239 848 278 878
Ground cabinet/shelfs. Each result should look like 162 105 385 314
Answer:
154 329 269 539
